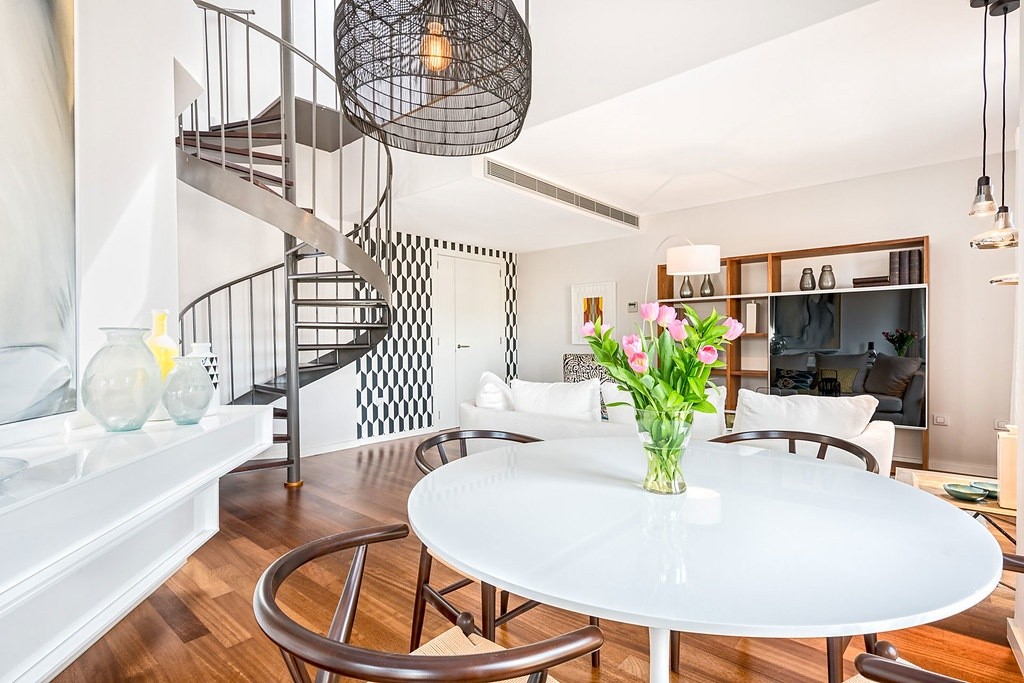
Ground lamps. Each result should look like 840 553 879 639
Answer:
970 0 1020 250
642 235 720 337
333 0 532 156
969 0 998 219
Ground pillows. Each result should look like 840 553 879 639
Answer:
476 371 879 438
770 352 923 398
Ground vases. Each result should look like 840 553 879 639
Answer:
635 408 694 495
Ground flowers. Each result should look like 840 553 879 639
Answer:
882 328 919 356
577 301 744 493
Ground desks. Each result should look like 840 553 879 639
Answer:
894 467 1016 545
406 438 1004 683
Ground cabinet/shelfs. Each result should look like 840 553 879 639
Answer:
657 235 930 428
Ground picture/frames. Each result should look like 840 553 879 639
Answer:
774 293 842 350
571 281 617 345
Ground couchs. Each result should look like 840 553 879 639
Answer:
459 397 895 479
771 365 925 426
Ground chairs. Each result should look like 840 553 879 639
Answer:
706 430 879 475
252 524 605 683
413 430 544 477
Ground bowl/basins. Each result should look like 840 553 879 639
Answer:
944 483 988 501
969 481 997 499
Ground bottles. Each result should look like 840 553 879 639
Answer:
183 344 220 417
800 268 815 291
818 265 835 290
142 309 178 424
81 328 163 432
163 357 214 427
700 274 714 297
680 276 693 298
770 333 787 355
